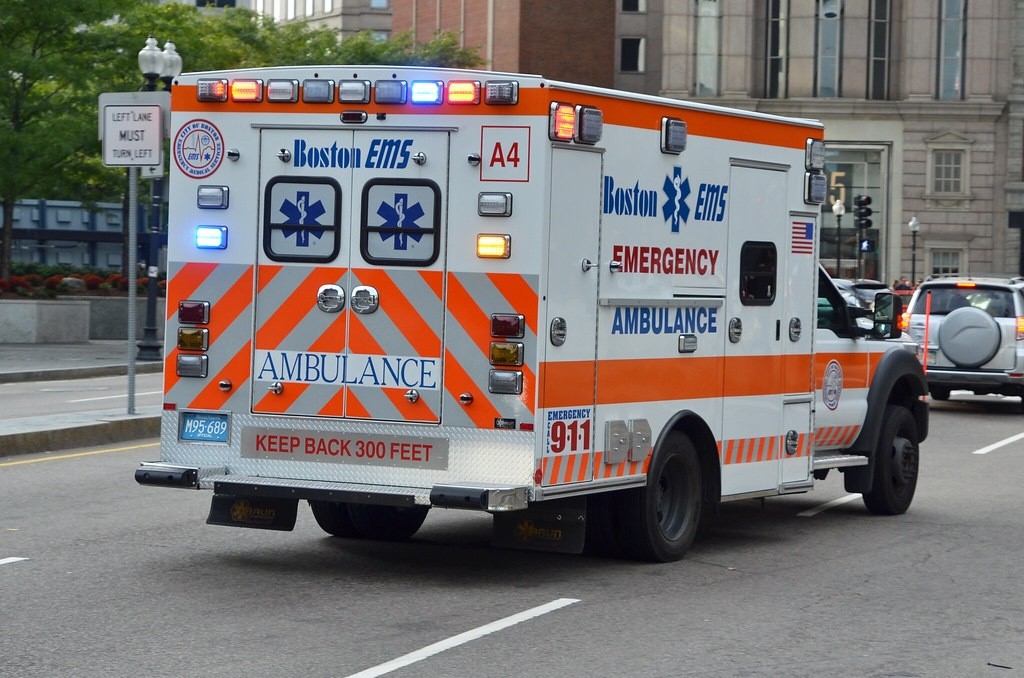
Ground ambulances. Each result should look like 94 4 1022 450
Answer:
136 66 929 563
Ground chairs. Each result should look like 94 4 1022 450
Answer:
948 295 971 309
987 299 1011 317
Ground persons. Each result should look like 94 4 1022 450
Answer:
889 276 924 291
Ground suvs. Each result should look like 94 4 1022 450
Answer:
900 273 1024 400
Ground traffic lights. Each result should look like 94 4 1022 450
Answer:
859 239 874 253
858 195 872 228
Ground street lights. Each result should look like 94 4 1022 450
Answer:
832 200 844 277
137 38 184 362
909 217 920 286
